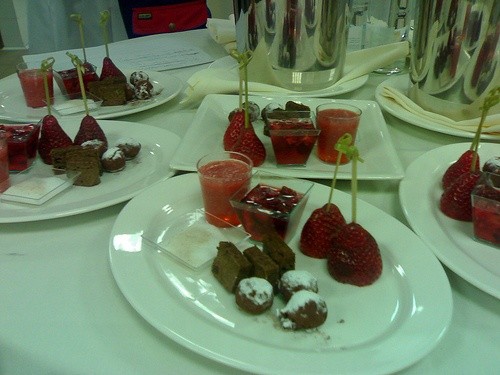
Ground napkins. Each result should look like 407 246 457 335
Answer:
177 42 410 110
381 75 500 134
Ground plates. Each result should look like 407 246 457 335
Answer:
0 164 81 205
399 141 500 298
41 90 103 115
208 55 369 97
169 93 404 181
0 68 181 123
374 73 500 140
109 171 453 375
0 117 179 222
140 208 252 274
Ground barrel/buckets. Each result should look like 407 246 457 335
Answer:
405 0 500 122
231 0 351 90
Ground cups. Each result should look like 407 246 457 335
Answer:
15 60 53 108
229 171 314 245
0 130 9 193
264 108 321 166
361 0 413 73
469 171 500 247
316 102 363 166
1 123 42 174
52 60 97 99
196 150 252 229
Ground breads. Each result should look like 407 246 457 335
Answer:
212 234 296 294
51 144 103 187
87 76 126 106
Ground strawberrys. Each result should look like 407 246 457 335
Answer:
37 115 108 164
224 112 266 167
300 203 382 287
440 150 483 221
82 56 126 89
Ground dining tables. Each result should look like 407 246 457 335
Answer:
0 24 499 375
